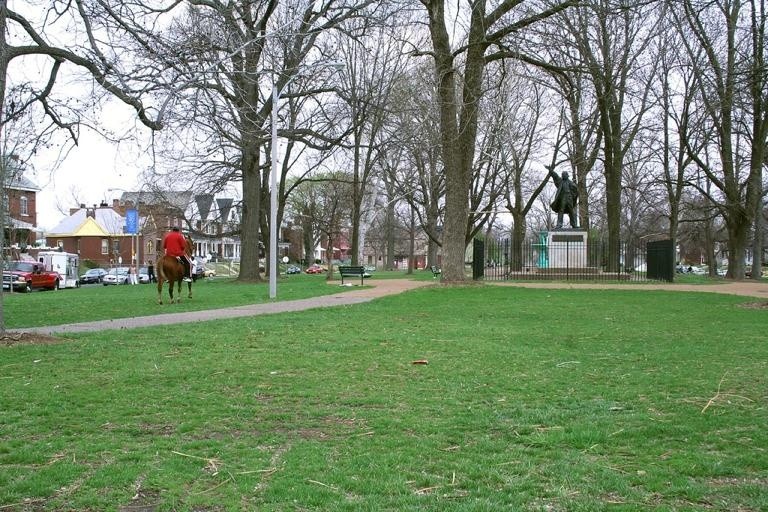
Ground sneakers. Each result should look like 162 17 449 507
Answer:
183 277 191 282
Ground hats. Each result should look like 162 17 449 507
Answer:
173 226 179 230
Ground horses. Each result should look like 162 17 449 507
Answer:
155 233 197 305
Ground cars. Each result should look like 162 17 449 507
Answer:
197 265 216 280
287 265 302 274
80 267 158 286
744 269 764 276
305 264 324 274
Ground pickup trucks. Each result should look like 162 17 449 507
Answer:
3 260 59 293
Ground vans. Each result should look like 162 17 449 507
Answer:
37 252 80 288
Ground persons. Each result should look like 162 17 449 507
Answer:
544 163 580 229
164 226 192 283
129 263 138 285
144 259 157 283
191 256 197 282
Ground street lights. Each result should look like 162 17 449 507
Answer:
269 61 347 299
107 187 140 267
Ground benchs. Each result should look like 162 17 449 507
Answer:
430 265 440 277
338 266 372 287
